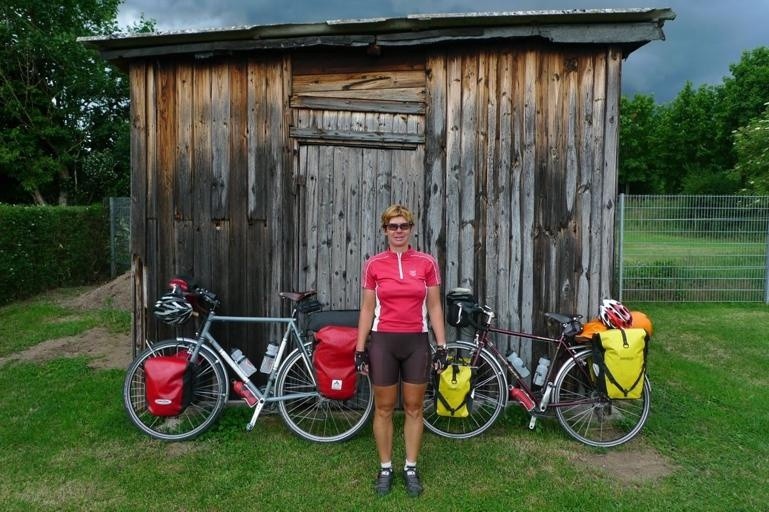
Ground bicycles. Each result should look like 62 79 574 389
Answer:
124 274 374 442
423 287 652 448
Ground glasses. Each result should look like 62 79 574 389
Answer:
384 224 414 229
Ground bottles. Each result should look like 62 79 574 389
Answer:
507 385 538 412
258 339 280 374
227 344 257 379
233 380 258 407
533 352 550 387
504 346 531 380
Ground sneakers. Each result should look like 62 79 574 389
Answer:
403 466 420 497
376 466 393 493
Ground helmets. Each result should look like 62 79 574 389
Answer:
153 295 193 326
600 299 633 329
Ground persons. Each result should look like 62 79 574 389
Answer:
353 204 449 499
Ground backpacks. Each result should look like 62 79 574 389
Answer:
144 358 191 417
592 328 649 400
312 326 360 399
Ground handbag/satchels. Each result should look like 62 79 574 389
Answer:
434 363 480 418
